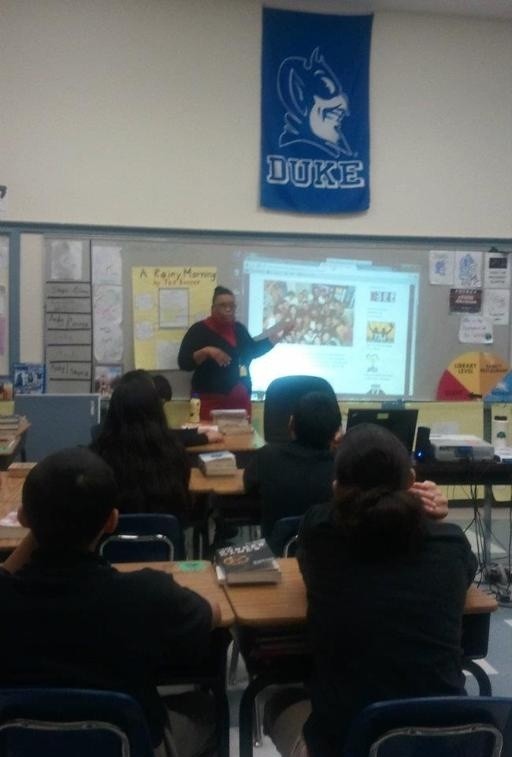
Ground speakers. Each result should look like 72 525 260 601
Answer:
413 425 431 465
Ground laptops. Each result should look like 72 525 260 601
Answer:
343 406 419 460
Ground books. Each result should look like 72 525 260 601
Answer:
0 382 31 456
180 408 254 479
212 538 284 586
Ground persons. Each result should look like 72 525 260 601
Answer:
176 286 296 427
262 420 478 757
241 390 343 542
85 368 193 561
262 278 353 346
0 447 222 757
150 373 239 550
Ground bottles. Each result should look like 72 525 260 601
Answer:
491 414 508 450
188 393 203 423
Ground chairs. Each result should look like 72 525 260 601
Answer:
263 375 342 442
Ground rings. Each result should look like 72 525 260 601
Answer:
431 500 437 510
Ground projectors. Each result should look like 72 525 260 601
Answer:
430 435 496 464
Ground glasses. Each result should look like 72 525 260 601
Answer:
215 301 237 311
